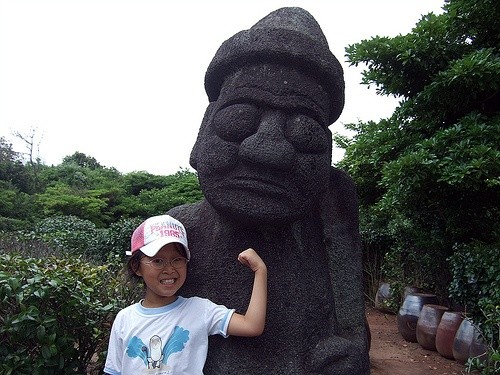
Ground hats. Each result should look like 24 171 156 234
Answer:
126 215 190 264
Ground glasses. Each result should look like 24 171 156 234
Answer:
140 257 190 270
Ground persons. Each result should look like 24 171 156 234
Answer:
102 213 269 375
162 7 372 375
